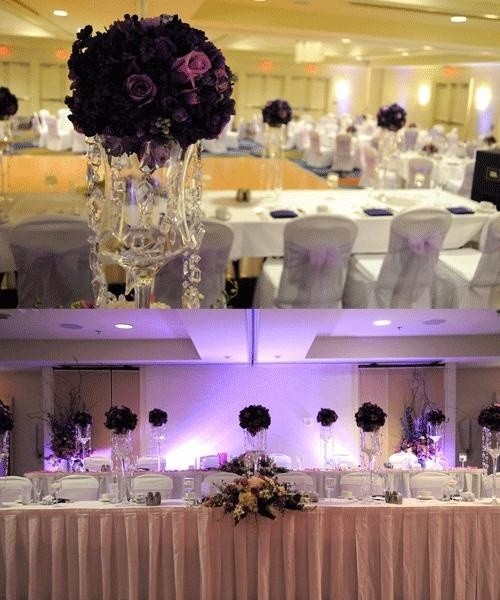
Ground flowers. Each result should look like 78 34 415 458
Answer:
316 400 387 433
63 9 238 170
73 402 168 437
478 403 500 431
193 475 319 526
238 403 270 436
376 102 407 133
263 98 293 130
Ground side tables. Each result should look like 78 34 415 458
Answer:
0 187 489 293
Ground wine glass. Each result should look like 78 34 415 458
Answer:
429 423 443 470
244 426 267 478
325 172 339 200
319 424 332 466
458 450 467 469
149 422 166 473
76 423 91 472
359 426 381 497
484 428 500 505
111 430 133 505
183 476 195 501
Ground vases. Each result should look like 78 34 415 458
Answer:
482 427 500 505
318 425 381 502
74 423 167 502
243 428 268 474
80 137 213 310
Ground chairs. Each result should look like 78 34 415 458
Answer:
291 111 498 194
7 205 499 309
0 451 500 502
30 108 71 154
